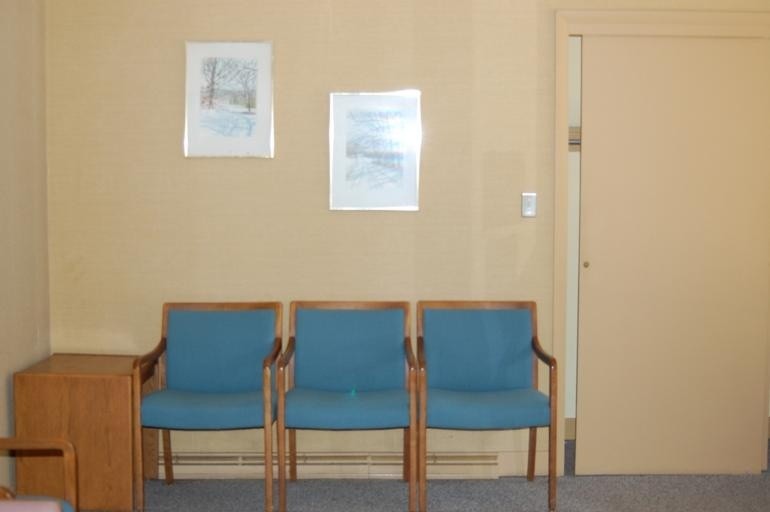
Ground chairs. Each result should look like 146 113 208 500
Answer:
274 297 420 511
132 297 285 511
415 296 559 512
0 435 81 512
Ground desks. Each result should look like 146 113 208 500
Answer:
8 350 159 512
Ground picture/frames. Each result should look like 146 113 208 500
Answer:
328 89 422 213
182 39 276 160
